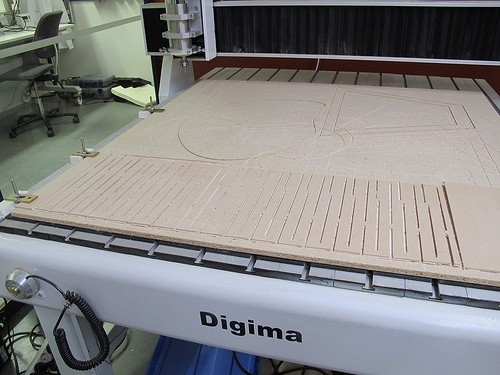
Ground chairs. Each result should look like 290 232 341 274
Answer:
0 10 82 138
